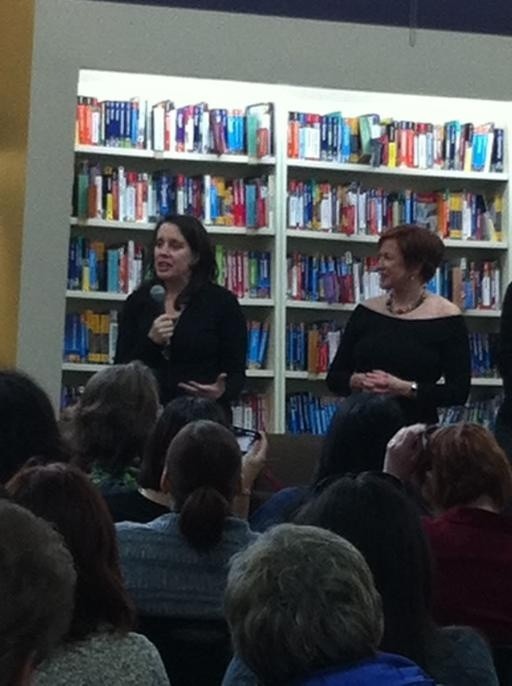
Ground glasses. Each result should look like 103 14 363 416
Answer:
312 468 405 506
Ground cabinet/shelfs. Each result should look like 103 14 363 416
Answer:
282 109 511 432
62 90 283 443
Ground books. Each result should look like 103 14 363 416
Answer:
211 242 270 436
287 110 509 245
69 93 277 233
59 233 159 414
285 249 387 436
424 256 505 437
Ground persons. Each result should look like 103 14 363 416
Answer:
111 215 248 427
1 357 512 684
325 222 473 425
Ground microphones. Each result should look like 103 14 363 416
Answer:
149 284 168 349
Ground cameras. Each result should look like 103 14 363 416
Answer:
235 426 262 453
